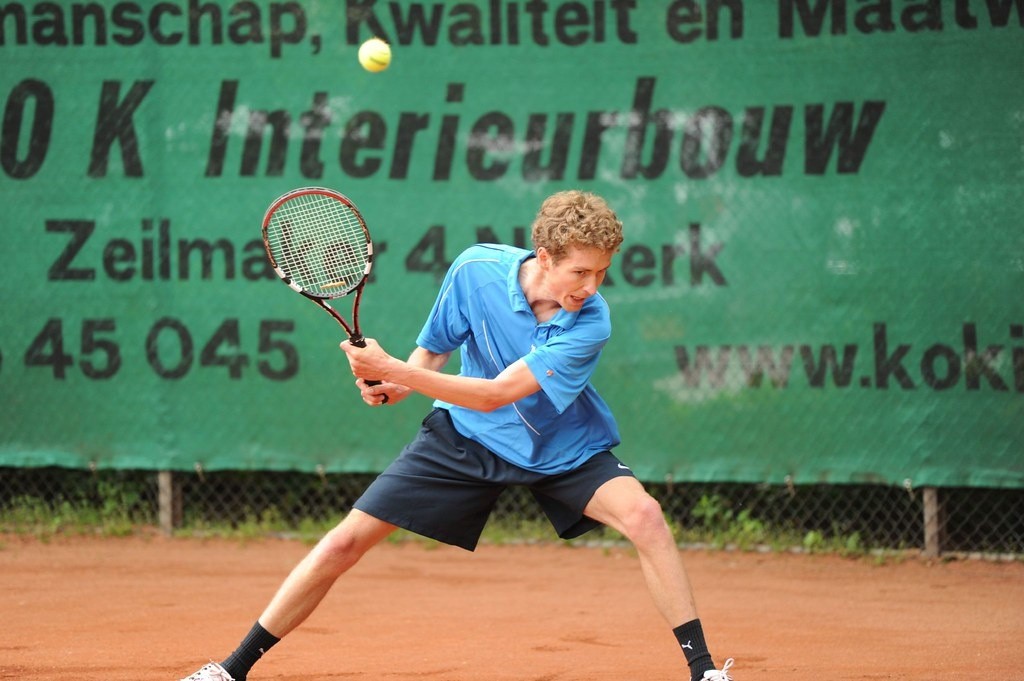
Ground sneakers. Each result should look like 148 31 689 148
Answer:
180 661 235 681
688 669 732 681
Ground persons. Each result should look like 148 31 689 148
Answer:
177 188 736 681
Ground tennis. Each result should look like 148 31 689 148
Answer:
357 38 392 74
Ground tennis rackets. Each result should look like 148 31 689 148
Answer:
262 184 392 406
